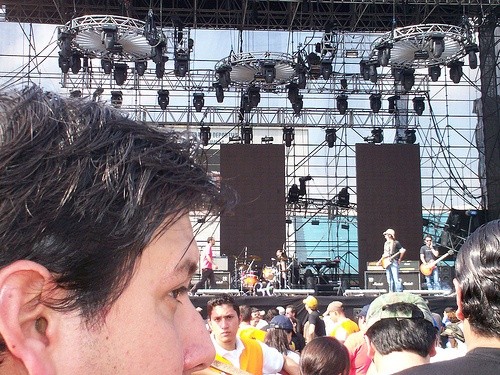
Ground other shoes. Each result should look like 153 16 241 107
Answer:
189 289 195 295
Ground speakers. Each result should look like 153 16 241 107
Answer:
205 255 231 289
364 271 420 290
315 284 339 296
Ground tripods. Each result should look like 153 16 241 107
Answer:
230 245 247 297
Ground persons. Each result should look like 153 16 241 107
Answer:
419 235 439 296
364 292 437 375
391 219 500 375
300 336 350 375
300 295 360 344
430 307 466 363
377 229 406 293
196 295 305 375
344 305 372 375
188 237 217 296
0 87 240 375
276 249 288 289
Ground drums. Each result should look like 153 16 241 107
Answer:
241 271 257 278
240 274 259 289
263 267 278 281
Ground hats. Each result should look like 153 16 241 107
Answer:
322 301 343 316
357 304 370 317
303 296 318 308
383 229 395 238
445 307 455 313
270 315 297 337
431 312 445 327
363 292 440 334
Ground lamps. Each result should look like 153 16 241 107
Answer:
57 0 482 209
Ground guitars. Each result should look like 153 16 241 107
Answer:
379 247 406 269
419 249 454 276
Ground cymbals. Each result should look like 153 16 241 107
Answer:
236 263 249 270
247 255 261 260
275 257 290 260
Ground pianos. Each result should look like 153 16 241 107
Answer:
300 259 340 284
